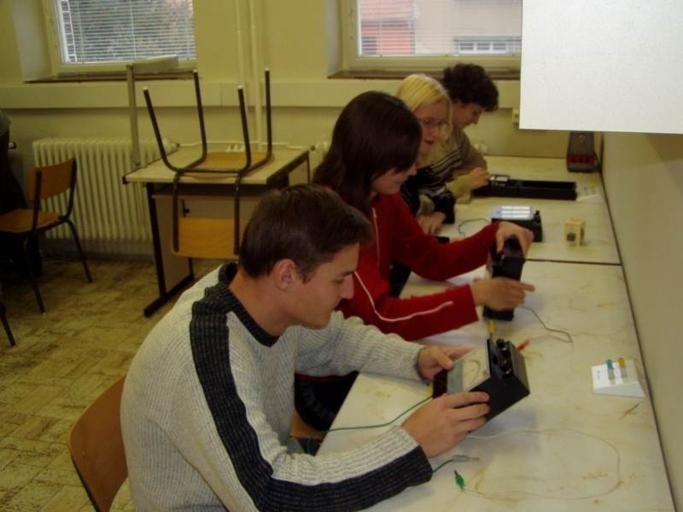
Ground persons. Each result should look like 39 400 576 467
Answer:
292 90 535 432
427 63 500 205
119 181 492 511
389 73 455 302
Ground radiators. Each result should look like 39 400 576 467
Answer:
30 137 287 243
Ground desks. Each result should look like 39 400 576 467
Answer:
315 154 673 512
121 145 312 317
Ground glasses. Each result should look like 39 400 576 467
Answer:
417 117 448 132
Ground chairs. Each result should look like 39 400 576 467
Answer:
0 156 93 313
66 374 125 510
143 70 274 262
